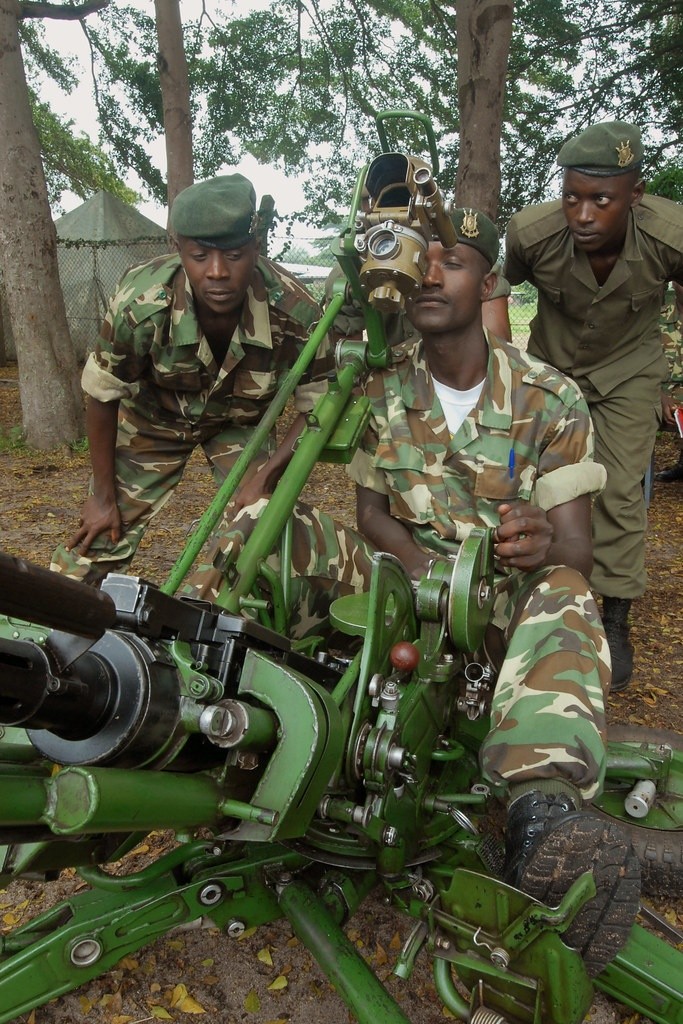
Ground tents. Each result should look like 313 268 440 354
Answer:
3 190 179 359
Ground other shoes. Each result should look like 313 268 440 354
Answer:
654 449 683 482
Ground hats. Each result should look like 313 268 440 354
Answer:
557 120 643 176
171 173 268 250
433 206 500 266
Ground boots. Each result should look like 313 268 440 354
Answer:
601 595 634 693
503 789 641 981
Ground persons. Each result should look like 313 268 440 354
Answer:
212 205 645 984
50 173 338 588
504 120 683 694
